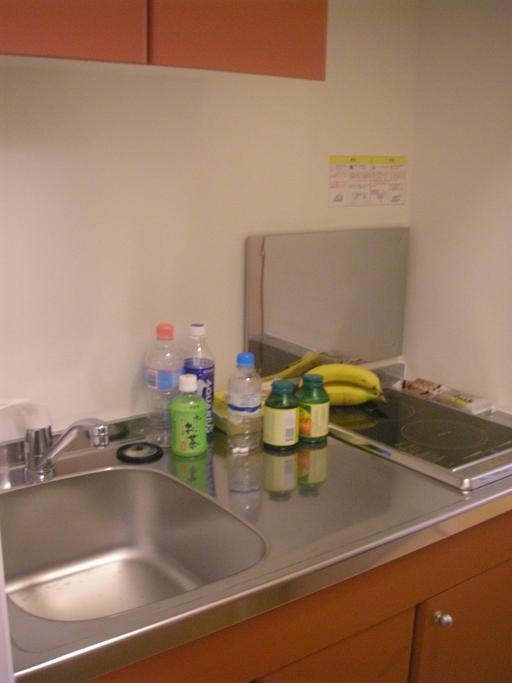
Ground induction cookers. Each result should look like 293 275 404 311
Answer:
294 386 512 491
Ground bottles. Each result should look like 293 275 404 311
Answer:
163 454 329 525
142 319 332 456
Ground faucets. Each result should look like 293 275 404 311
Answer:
22 415 110 479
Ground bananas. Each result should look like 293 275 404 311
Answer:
297 363 386 407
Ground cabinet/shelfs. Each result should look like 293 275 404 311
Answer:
92 555 415 682
411 508 512 681
0 0 330 87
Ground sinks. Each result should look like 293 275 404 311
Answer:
2 466 269 624
